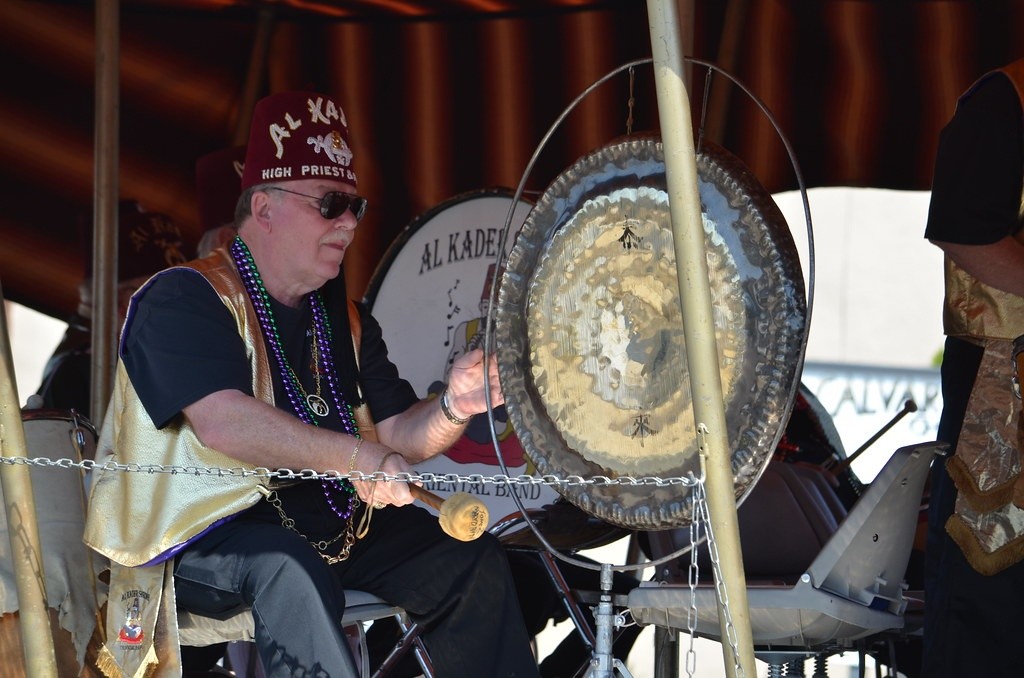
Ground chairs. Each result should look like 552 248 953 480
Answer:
626 439 951 678
81 472 437 678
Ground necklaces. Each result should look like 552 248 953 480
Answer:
228 236 362 518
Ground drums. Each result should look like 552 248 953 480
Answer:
360 189 568 540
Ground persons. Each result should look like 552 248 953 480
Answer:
33 197 188 422
916 58 1024 677
193 148 252 259
79 93 542 678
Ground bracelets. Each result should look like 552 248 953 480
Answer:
440 387 474 425
348 439 364 471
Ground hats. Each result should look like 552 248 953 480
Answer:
239 91 358 194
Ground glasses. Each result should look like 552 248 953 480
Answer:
261 186 368 223
